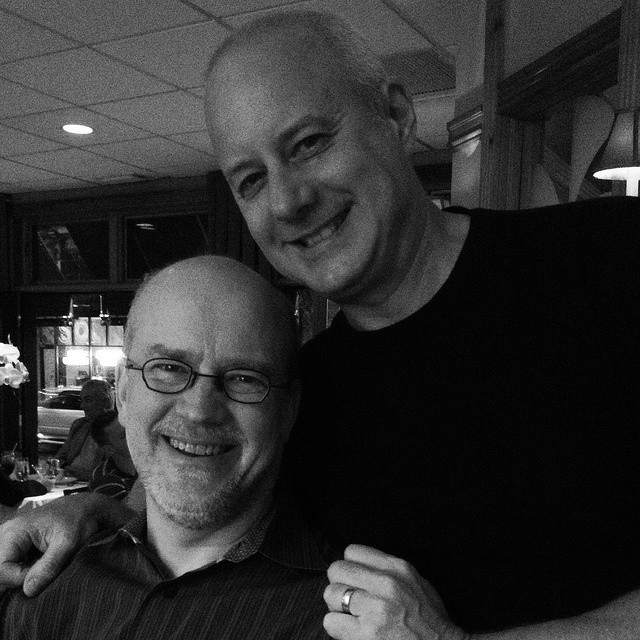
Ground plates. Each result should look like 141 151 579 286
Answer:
57 476 76 483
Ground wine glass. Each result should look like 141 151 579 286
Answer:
45 459 59 492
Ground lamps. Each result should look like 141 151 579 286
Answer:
593 111 640 198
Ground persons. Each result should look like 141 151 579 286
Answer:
0 255 344 640
1 9 639 640
87 410 146 512
55 379 115 481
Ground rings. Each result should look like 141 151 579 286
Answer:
341 587 356 615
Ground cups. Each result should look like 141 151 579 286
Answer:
13 457 29 480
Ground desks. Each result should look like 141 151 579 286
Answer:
0 473 89 523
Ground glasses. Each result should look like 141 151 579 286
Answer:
125 357 290 405
